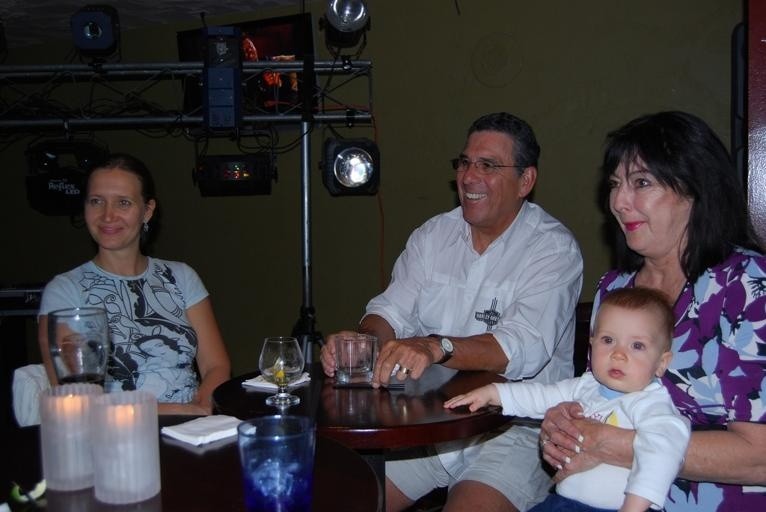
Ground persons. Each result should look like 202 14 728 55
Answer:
36 149 234 425
317 107 589 510
519 103 764 510
439 283 692 510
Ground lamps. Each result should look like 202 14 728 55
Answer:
316 1 380 66
64 2 122 65
192 150 276 198
29 119 100 176
322 132 381 200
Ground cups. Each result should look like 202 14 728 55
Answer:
237 415 317 512
48 307 110 388
334 334 377 383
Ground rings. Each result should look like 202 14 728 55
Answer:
399 365 411 375
538 437 551 450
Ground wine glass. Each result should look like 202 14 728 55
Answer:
259 336 305 405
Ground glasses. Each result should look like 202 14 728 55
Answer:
450 158 524 174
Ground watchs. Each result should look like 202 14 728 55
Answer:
430 330 453 365
